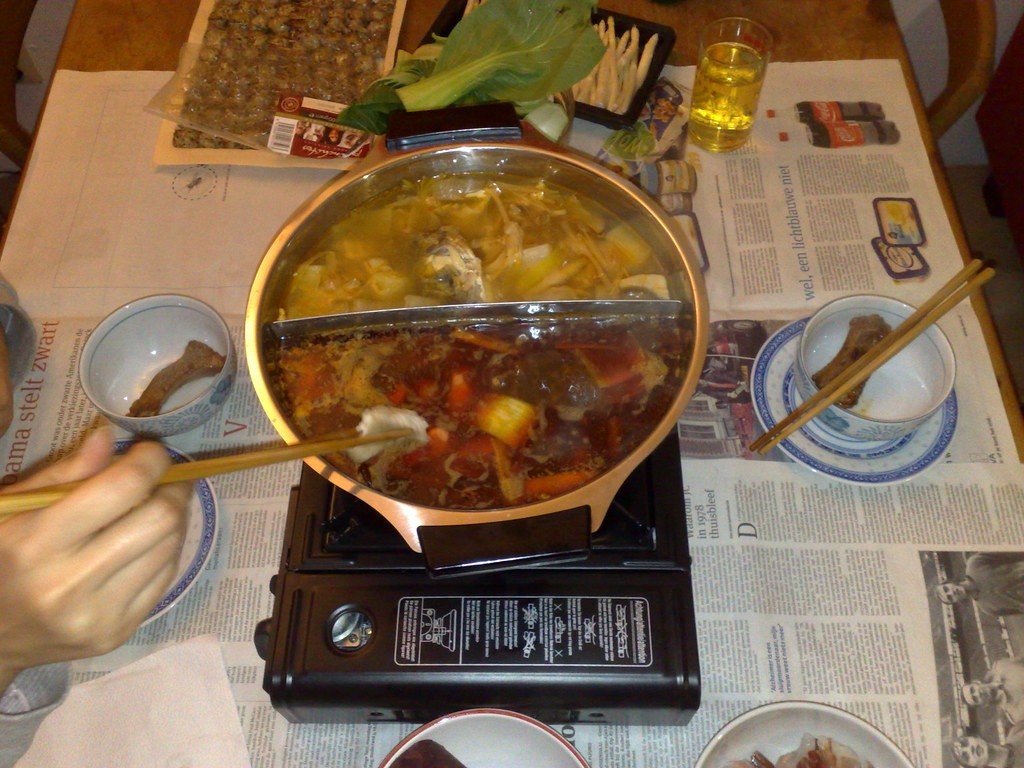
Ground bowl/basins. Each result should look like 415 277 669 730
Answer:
378 708 592 768
79 293 237 437
794 294 957 442
693 700 915 768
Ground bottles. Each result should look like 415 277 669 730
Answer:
766 101 885 124
778 121 900 148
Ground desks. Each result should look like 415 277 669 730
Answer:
0 0 1024 768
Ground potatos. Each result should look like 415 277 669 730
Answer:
333 0 607 134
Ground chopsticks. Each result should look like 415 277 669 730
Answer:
749 258 995 454
0 427 414 520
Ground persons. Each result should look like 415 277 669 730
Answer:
0 331 191 702
961 655 1024 725
297 120 357 148
951 724 1024 768
934 551 1023 615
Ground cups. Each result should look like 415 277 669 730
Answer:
688 17 773 151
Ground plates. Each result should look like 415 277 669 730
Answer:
750 316 959 487
56 439 218 628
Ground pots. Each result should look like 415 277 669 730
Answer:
243 102 710 579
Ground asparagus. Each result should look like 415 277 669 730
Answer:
568 15 661 114
